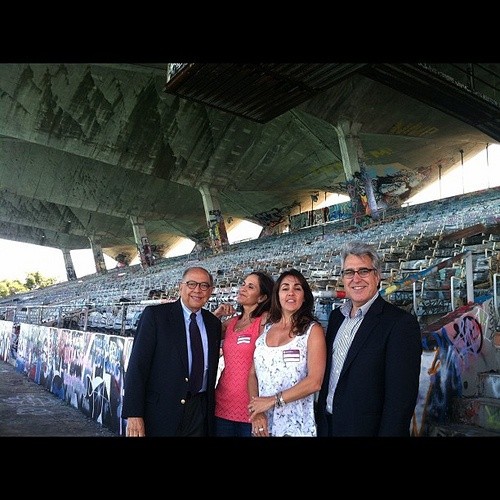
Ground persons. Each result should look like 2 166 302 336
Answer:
314 241 421 436
211 272 276 437
121 267 221 438
247 269 327 436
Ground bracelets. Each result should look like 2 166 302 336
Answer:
275 391 285 409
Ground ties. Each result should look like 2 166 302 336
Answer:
189 313 204 395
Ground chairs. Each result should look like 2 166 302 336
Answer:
0 187 500 336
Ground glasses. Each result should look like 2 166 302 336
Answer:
342 267 378 278
181 280 212 291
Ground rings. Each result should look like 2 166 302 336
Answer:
259 427 264 432
